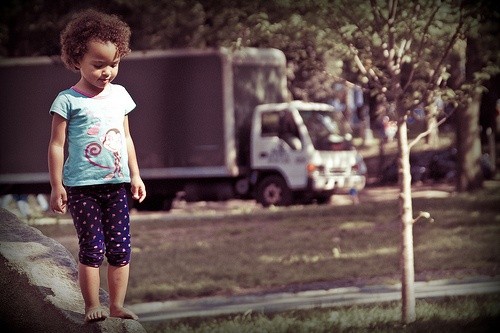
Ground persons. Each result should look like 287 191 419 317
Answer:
46 8 147 324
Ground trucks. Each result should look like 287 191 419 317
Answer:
0 47 368 212
